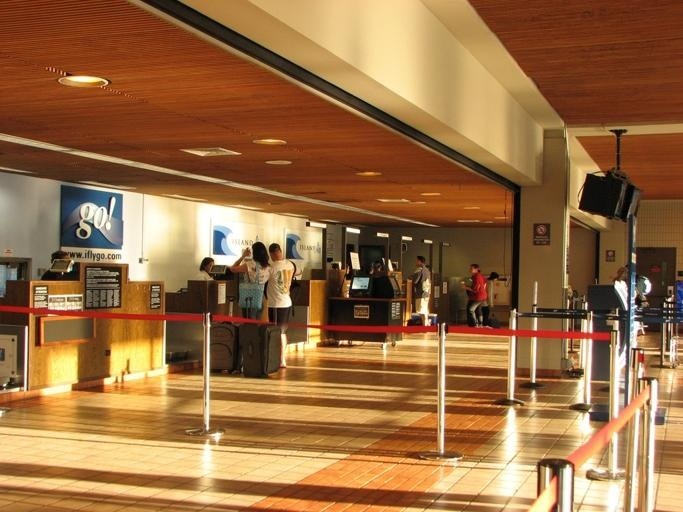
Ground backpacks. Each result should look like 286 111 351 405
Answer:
636 275 651 295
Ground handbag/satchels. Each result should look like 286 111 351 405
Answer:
239 282 264 309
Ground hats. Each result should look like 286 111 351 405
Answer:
487 272 499 279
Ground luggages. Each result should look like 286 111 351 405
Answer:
210 295 281 378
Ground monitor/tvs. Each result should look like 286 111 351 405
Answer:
579 174 630 221
620 186 642 222
375 276 400 293
350 275 371 297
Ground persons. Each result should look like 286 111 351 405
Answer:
612 266 628 298
263 242 301 368
196 256 214 281
407 255 431 327
634 273 645 336
229 241 271 320
50 250 69 264
458 264 499 330
